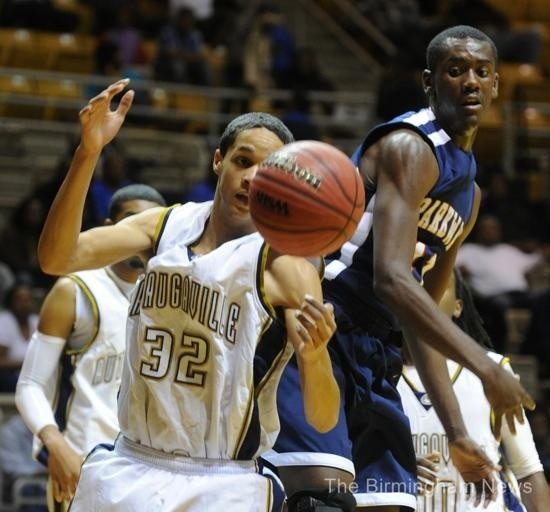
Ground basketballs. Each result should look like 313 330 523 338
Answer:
249 140 365 257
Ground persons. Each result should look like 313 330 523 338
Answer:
392 262 549 511
23 0 318 96
14 183 168 510
1 281 41 393
37 77 341 512
264 24 537 511
449 145 533 211
455 209 549 357
